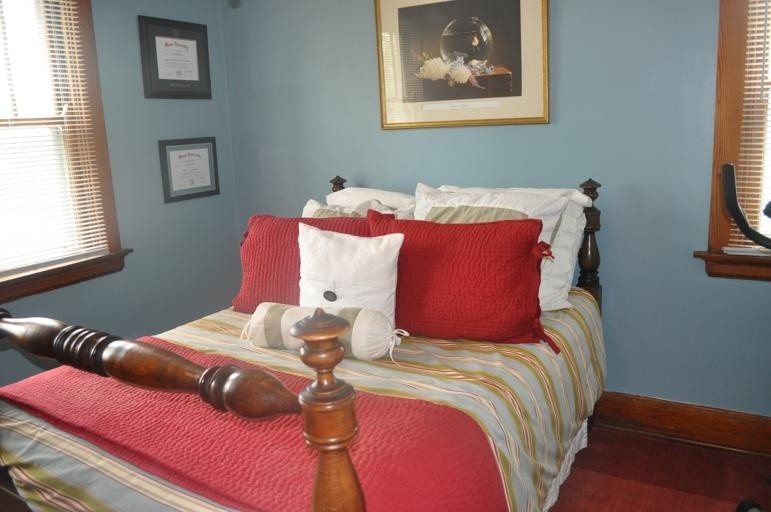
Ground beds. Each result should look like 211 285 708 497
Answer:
1 172 612 512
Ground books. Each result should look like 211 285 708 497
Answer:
722 244 771 256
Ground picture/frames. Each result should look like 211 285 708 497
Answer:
158 135 221 205
138 13 213 101
373 0 552 131
